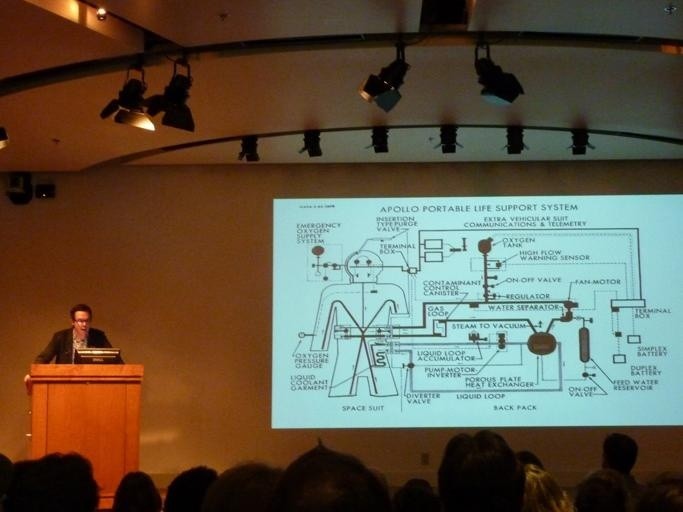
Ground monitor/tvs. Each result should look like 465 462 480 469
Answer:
74 348 121 363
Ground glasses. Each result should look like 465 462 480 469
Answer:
73 319 88 323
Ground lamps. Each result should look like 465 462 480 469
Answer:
360 42 524 112
100 56 197 133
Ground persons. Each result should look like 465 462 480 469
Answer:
273 436 384 511
438 430 526 512
24 303 125 380
574 468 633 512
200 460 280 511
639 470 683 512
0 449 100 512
163 466 219 512
601 433 645 500
512 452 544 471
111 471 162 512
522 463 574 512
391 486 441 512
